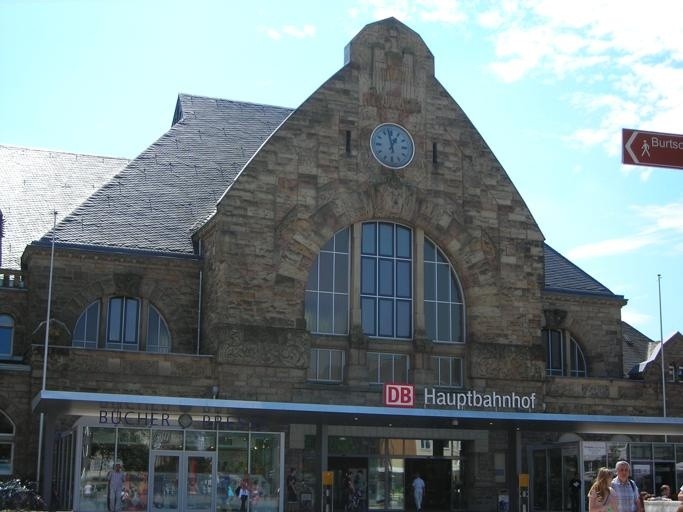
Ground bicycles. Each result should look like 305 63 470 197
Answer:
0 474 50 512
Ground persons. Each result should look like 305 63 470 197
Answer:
568 473 581 512
223 479 234 512
641 484 683 512
610 461 644 512
412 473 426 512
587 467 618 512
287 467 297 502
107 464 125 512
238 484 248 512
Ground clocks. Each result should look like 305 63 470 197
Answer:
369 120 416 169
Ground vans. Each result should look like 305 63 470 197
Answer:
633 464 650 479
